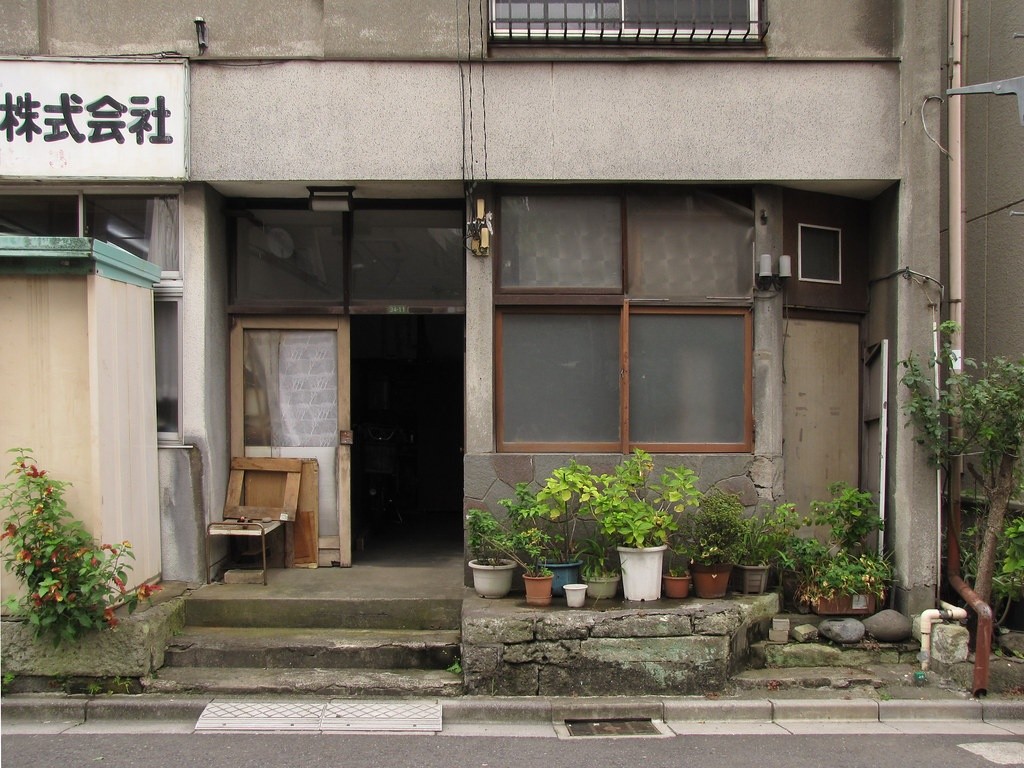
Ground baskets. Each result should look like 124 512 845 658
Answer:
362 441 398 474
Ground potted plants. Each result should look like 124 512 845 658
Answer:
466 447 901 616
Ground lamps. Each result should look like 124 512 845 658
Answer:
757 253 792 285
305 185 356 212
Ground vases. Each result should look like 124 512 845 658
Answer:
811 585 887 613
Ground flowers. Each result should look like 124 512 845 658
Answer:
795 546 899 602
